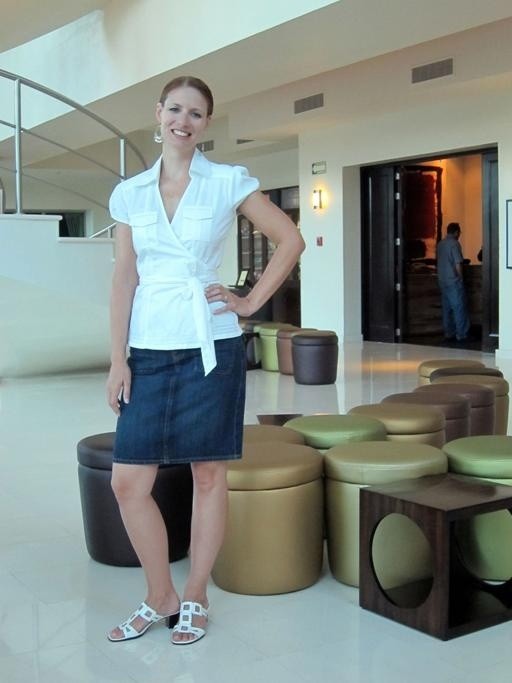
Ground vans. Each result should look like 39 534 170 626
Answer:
168 597 210 645
107 600 180 642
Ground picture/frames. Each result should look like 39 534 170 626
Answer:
505 198 512 269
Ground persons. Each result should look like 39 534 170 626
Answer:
477 247 483 261
436 223 475 342
104 75 308 647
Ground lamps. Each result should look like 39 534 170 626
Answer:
313 189 321 209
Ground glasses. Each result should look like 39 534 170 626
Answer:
313 189 321 209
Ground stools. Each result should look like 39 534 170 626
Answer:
76 433 193 567
210 444 324 596
284 414 387 455
346 359 509 450
239 320 317 374
324 441 448 589
291 330 338 385
243 424 304 444
443 435 512 582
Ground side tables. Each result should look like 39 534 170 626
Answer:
359 472 511 641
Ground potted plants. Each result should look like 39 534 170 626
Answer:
505 198 512 269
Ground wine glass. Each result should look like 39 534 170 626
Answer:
224 296 228 301
